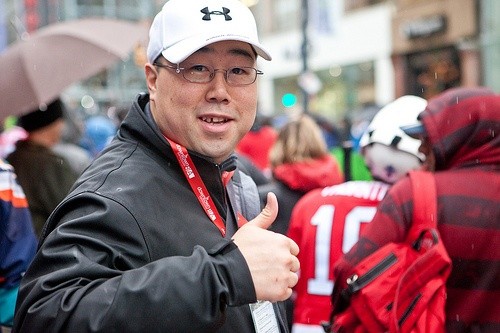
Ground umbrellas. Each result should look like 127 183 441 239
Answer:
0 17 149 128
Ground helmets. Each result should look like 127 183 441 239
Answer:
358 95 428 186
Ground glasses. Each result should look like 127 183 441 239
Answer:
152 63 264 86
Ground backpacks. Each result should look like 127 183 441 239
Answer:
329 170 452 333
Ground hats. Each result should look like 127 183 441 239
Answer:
399 125 428 140
16 98 63 132
146 0 272 65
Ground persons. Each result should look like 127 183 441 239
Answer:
11 0 301 333
286 96 423 333
0 95 130 333
235 112 388 235
321 87 500 333
